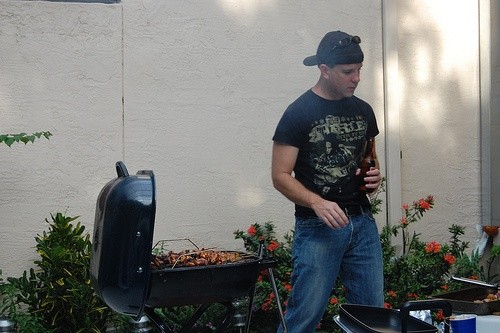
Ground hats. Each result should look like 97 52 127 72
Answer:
303 31 366 66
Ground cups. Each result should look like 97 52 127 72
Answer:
450 315 477 333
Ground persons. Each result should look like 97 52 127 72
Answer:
271 30 387 333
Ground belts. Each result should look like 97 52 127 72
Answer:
294 204 372 217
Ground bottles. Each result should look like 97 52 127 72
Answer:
357 138 376 195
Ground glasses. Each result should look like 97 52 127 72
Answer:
328 36 362 58
